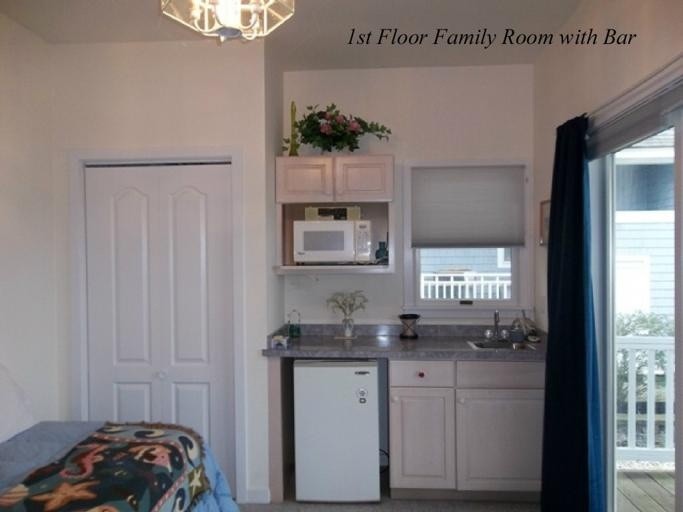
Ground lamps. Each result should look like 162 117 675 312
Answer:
160 0 294 44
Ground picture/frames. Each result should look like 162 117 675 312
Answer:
539 200 551 245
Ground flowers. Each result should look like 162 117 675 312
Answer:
291 102 392 152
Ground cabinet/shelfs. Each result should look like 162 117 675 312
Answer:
456 360 546 491
275 154 396 275
388 361 455 491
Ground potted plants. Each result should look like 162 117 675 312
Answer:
325 288 370 337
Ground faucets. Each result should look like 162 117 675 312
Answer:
494 310 501 341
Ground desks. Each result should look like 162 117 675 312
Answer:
0 419 240 512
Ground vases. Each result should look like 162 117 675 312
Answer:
321 147 332 157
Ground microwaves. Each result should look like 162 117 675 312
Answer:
291 220 371 262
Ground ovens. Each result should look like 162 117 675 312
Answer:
292 358 380 503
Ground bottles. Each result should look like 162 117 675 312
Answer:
287 310 302 337
375 242 387 265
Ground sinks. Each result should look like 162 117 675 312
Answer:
473 340 537 352
